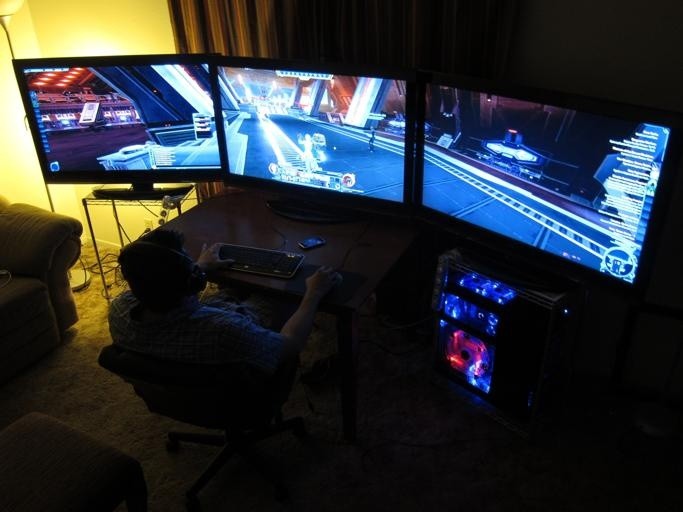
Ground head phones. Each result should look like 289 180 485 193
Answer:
121 244 208 294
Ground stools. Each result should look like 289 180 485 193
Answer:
1 412 150 512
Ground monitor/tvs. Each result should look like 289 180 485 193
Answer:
12 52 219 200
414 71 678 299
213 58 413 223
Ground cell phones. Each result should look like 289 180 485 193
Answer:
298 235 326 249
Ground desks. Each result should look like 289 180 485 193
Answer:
123 188 425 431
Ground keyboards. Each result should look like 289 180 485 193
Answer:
219 243 305 279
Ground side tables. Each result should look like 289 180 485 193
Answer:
82 185 201 305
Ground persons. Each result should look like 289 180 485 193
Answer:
117 226 339 383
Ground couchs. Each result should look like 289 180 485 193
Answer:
0 195 83 379
